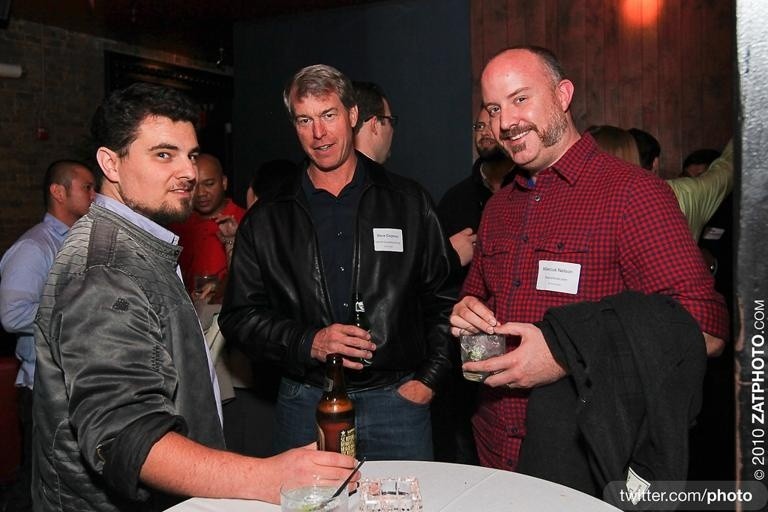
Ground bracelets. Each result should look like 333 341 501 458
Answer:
223 238 238 246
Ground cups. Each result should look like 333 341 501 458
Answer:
356 477 422 512
460 331 505 381
280 478 348 512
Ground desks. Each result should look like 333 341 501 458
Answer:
162 459 627 512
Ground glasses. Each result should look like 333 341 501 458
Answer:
363 113 399 126
471 121 491 131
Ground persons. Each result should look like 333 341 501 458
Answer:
218 63 460 462
435 102 516 465
216 153 306 458
30 83 360 512
450 45 727 512
681 149 734 304
0 160 99 512
588 124 641 166
353 79 477 268
627 129 734 245
167 151 246 303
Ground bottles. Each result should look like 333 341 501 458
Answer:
314 353 356 496
349 292 377 371
